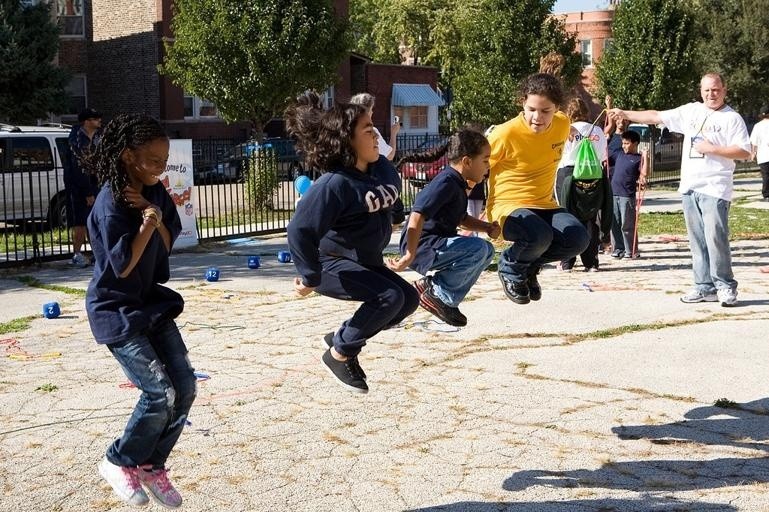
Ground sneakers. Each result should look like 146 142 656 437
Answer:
99 456 182 509
321 332 368 393
413 275 467 326
680 288 738 307
498 271 541 304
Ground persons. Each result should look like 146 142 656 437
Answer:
555 96 608 274
610 71 754 310
87 112 200 509
63 105 107 272
599 93 631 255
747 104 769 202
605 130 651 261
466 179 486 239
385 129 503 326
347 90 404 165
279 89 422 396
464 48 588 305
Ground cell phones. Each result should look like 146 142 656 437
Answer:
395 116 399 124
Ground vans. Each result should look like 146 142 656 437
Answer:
627 124 684 165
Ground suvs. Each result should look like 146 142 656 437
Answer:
193 135 304 182
0 119 75 234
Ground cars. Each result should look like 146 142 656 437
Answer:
194 164 217 180
400 136 450 190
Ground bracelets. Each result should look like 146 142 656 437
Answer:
143 215 160 227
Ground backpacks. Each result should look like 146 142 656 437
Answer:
572 138 603 180
